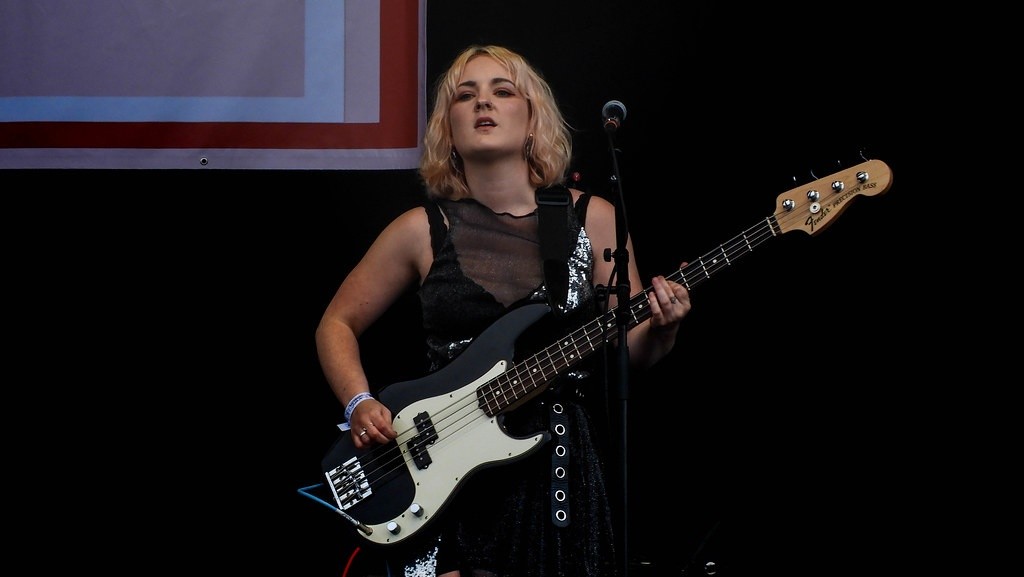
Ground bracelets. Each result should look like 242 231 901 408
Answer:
342 392 375 426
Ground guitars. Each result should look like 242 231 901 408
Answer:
316 155 897 548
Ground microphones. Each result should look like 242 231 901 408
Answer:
602 100 627 133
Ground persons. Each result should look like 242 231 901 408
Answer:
314 46 693 577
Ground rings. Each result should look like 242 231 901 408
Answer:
670 296 677 304
359 428 367 438
